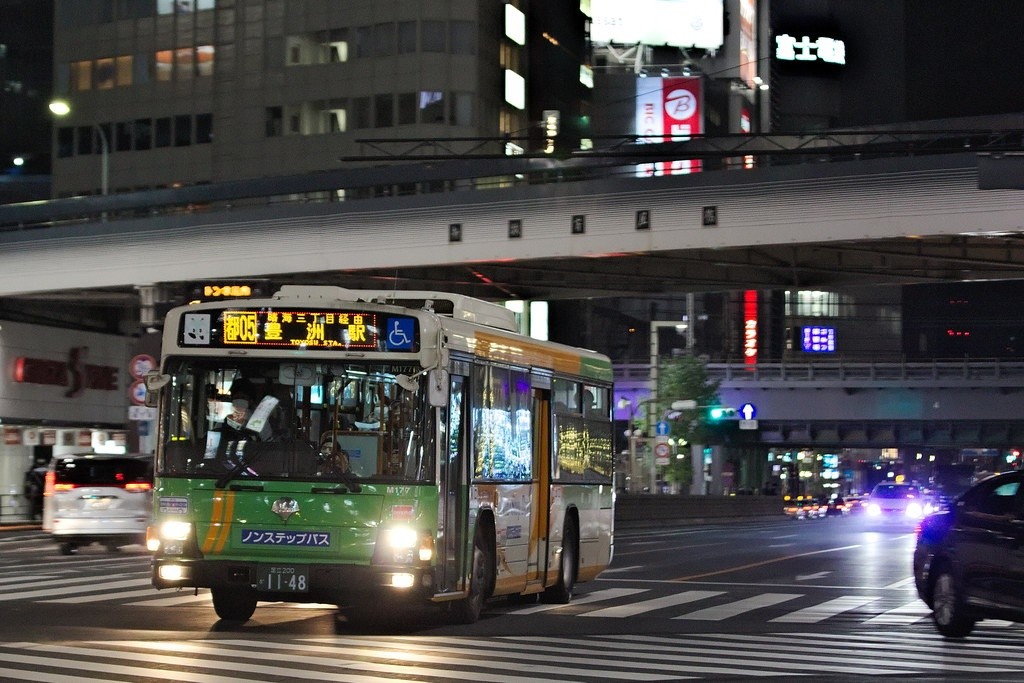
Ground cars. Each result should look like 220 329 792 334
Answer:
913 470 1024 637
781 480 951 526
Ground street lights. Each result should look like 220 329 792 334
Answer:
48 96 110 221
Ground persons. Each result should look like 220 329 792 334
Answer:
24 452 47 520
374 396 389 419
574 390 594 410
321 437 361 477
222 378 279 441
330 415 349 430
976 464 993 479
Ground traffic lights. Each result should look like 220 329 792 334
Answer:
1008 450 1018 468
710 407 744 421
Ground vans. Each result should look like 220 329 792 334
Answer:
44 452 154 554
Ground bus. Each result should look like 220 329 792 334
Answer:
146 280 618 623
